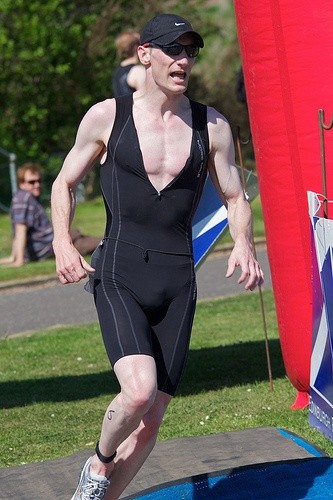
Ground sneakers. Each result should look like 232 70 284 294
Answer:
70 457 115 500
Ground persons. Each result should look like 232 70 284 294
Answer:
50 12 265 500
113 31 146 98
0 162 102 268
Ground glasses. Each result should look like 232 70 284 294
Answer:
142 42 199 58
23 180 40 184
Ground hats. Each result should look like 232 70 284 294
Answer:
139 15 204 49
